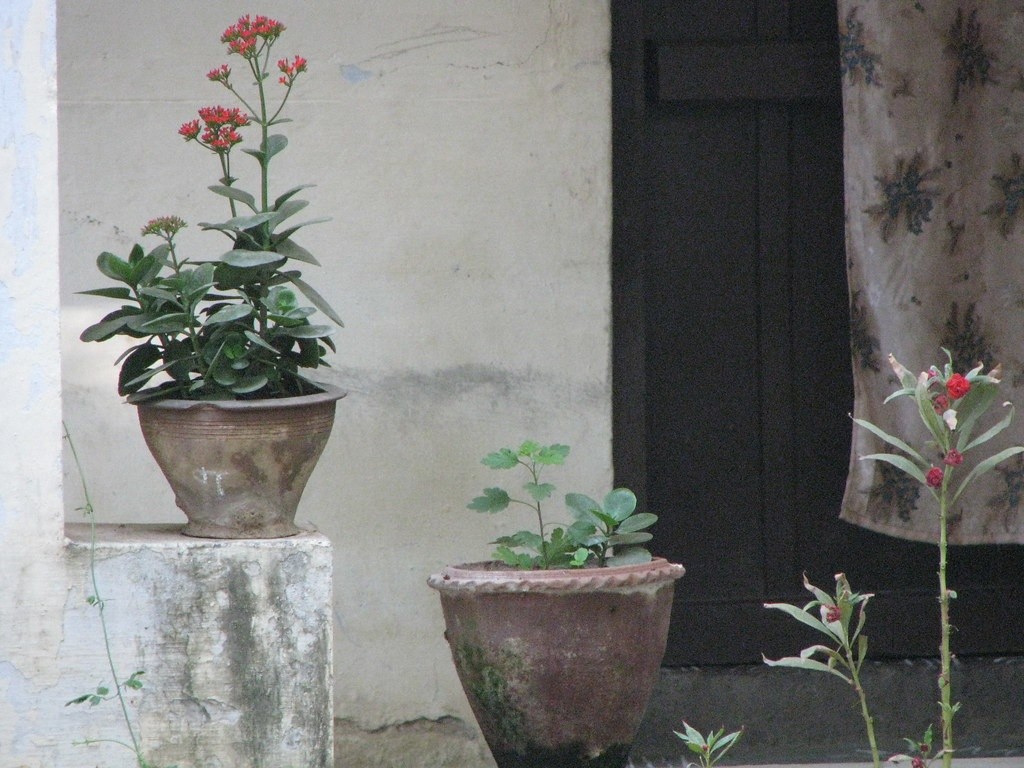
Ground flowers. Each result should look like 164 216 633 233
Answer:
672 720 746 768
887 722 958 768
759 568 883 768
845 344 1024 768
75 13 344 401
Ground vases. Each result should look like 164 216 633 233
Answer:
128 376 348 541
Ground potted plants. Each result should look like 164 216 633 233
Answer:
424 438 689 768
